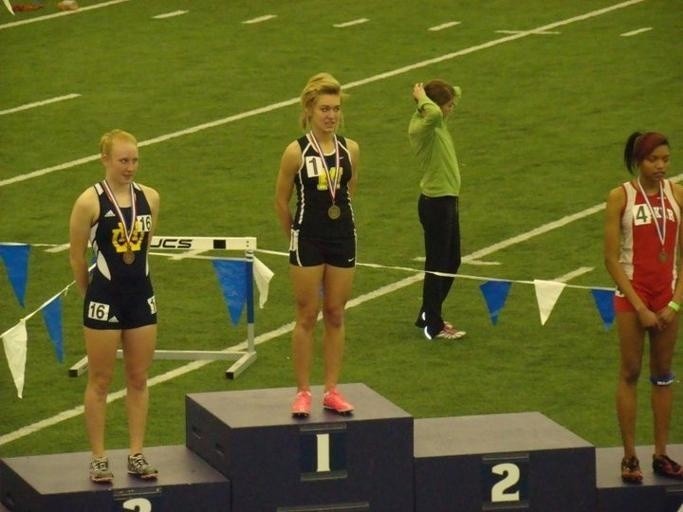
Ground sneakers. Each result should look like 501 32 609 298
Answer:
322 390 354 413
443 321 453 330
622 456 644 482
87 456 114 482
434 326 467 340
291 390 312 418
127 452 159 479
652 453 683 481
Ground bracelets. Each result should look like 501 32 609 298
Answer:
666 301 680 313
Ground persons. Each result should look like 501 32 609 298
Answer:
604 131 681 483
274 74 360 419
69 128 160 482
407 80 469 339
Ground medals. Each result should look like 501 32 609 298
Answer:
328 205 341 222
657 251 669 265
121 248 137 265
